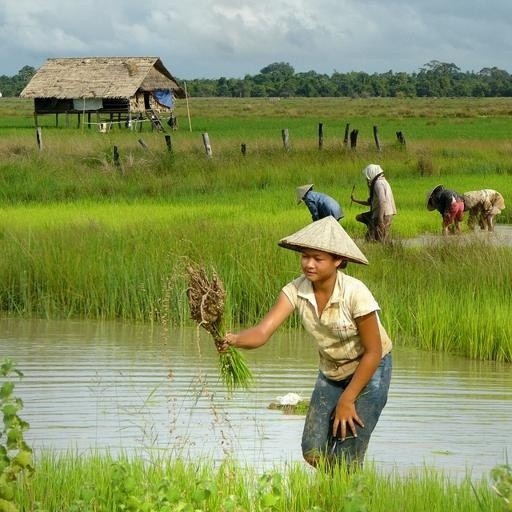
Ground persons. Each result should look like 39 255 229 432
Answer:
351 164 397 244
215 215 393 477
293 183 344 221
426 184 465 235
461 189 506 231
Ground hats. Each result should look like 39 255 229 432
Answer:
297 184 313 204
426 184 443 211
278 215 368 265
